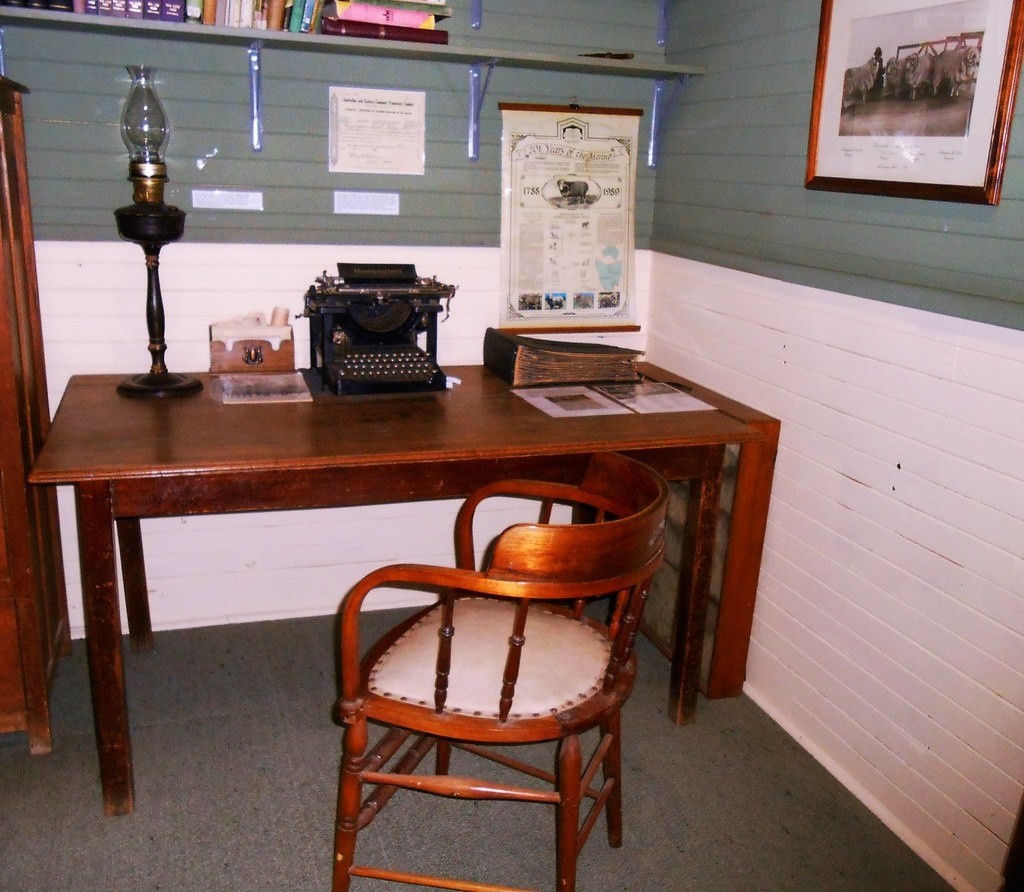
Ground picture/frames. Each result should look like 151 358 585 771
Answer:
803 0 1024 206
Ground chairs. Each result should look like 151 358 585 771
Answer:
328 451 676 892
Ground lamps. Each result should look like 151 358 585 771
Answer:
114 65 206 403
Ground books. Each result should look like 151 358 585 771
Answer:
483 328 645 387
0 0 451 45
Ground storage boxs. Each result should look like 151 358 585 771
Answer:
208 325 295 376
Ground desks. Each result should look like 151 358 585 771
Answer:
31 361 780 820
